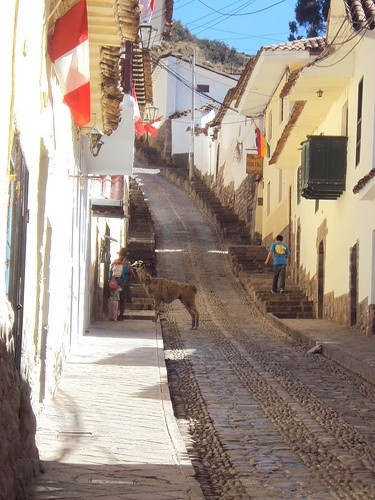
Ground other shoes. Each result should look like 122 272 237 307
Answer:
119 315 123 320
271 289 274 294
280 289 284 293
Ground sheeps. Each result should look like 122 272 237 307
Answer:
128 258 202 332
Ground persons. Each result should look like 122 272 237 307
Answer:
265 235 290 293
108 286 122 320
110 248 135 317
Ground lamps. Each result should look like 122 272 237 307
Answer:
78 111 105 158
317 88 324 97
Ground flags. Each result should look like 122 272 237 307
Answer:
257 129 270 158
48 0 90 127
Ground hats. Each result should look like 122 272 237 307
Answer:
118 248 130 255
108 280 118 291
